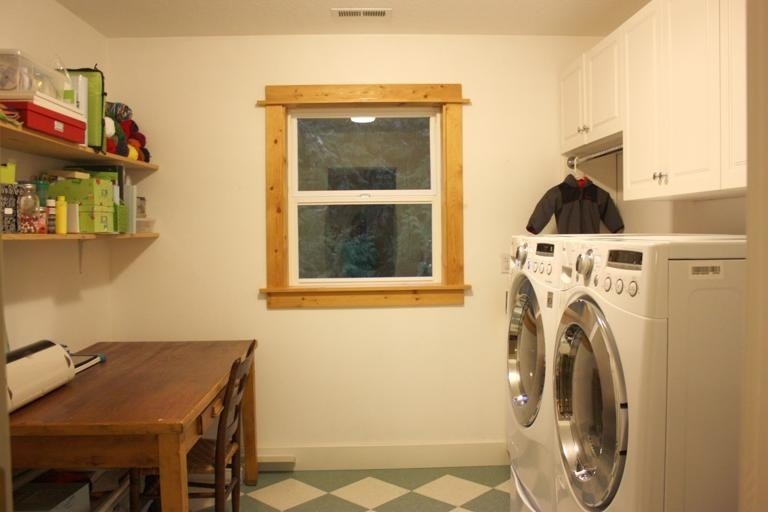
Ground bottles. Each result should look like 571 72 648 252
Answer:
17 180 67 235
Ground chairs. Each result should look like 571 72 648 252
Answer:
122 340 260 511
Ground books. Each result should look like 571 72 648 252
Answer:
69 354 101 374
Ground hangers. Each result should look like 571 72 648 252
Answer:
568 157 587 189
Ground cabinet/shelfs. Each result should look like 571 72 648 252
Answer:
622 0 767 206
554 24 626 162
0 112 164 246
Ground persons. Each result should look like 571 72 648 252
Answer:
316 173 423 278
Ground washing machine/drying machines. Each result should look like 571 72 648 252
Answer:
546 233 748 512
503 235 553 511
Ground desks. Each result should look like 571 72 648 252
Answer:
2 333 263 511
